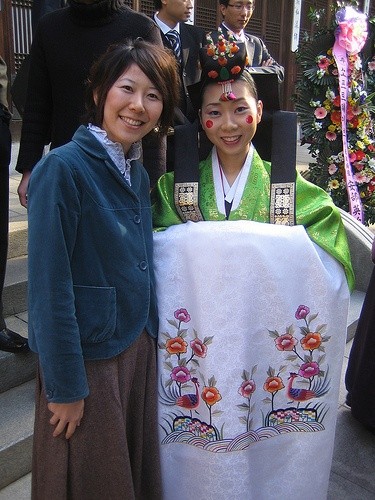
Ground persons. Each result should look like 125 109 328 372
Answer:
26 37 182 500
150 0 207 125
10 0 168 210
0 56 28 357
206 0 285 86
153 27 356 500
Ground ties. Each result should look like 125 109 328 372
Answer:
166 29 182 64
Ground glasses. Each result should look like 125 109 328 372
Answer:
228 4 255 11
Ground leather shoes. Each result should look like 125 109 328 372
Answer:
0 328 28 352
